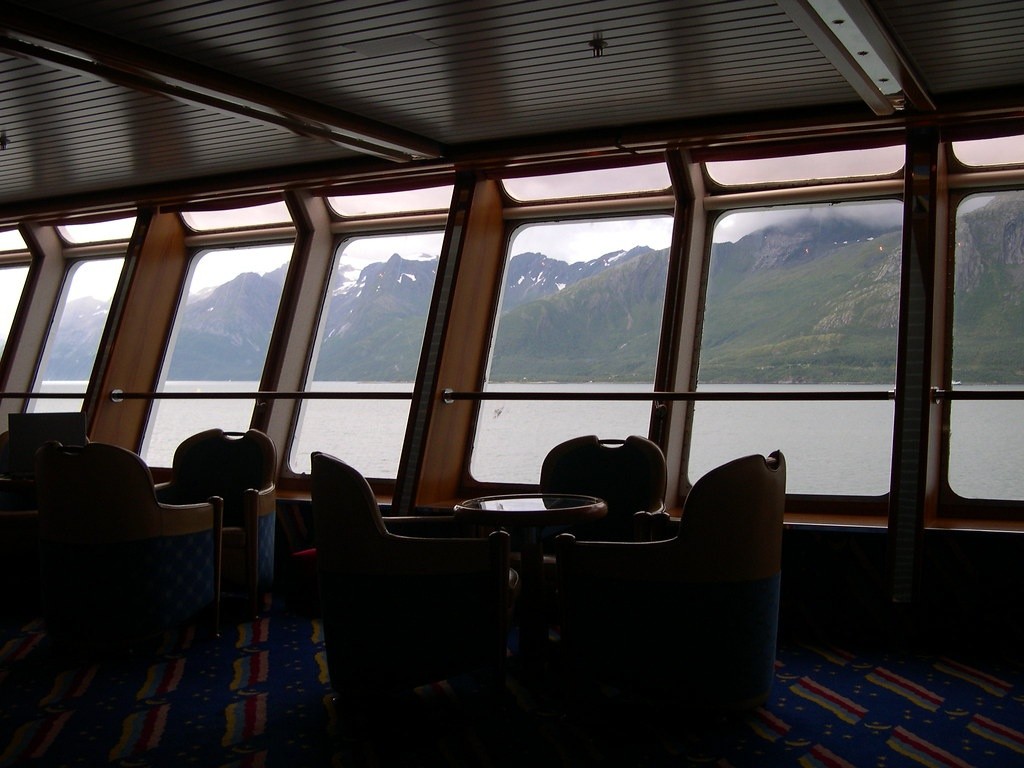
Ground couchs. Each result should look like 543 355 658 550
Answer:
153 427 278 621
35 439 216 653
535 436 669 571
566 451 785 720
310 449 511 708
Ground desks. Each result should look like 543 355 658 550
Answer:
454 494 608 716
